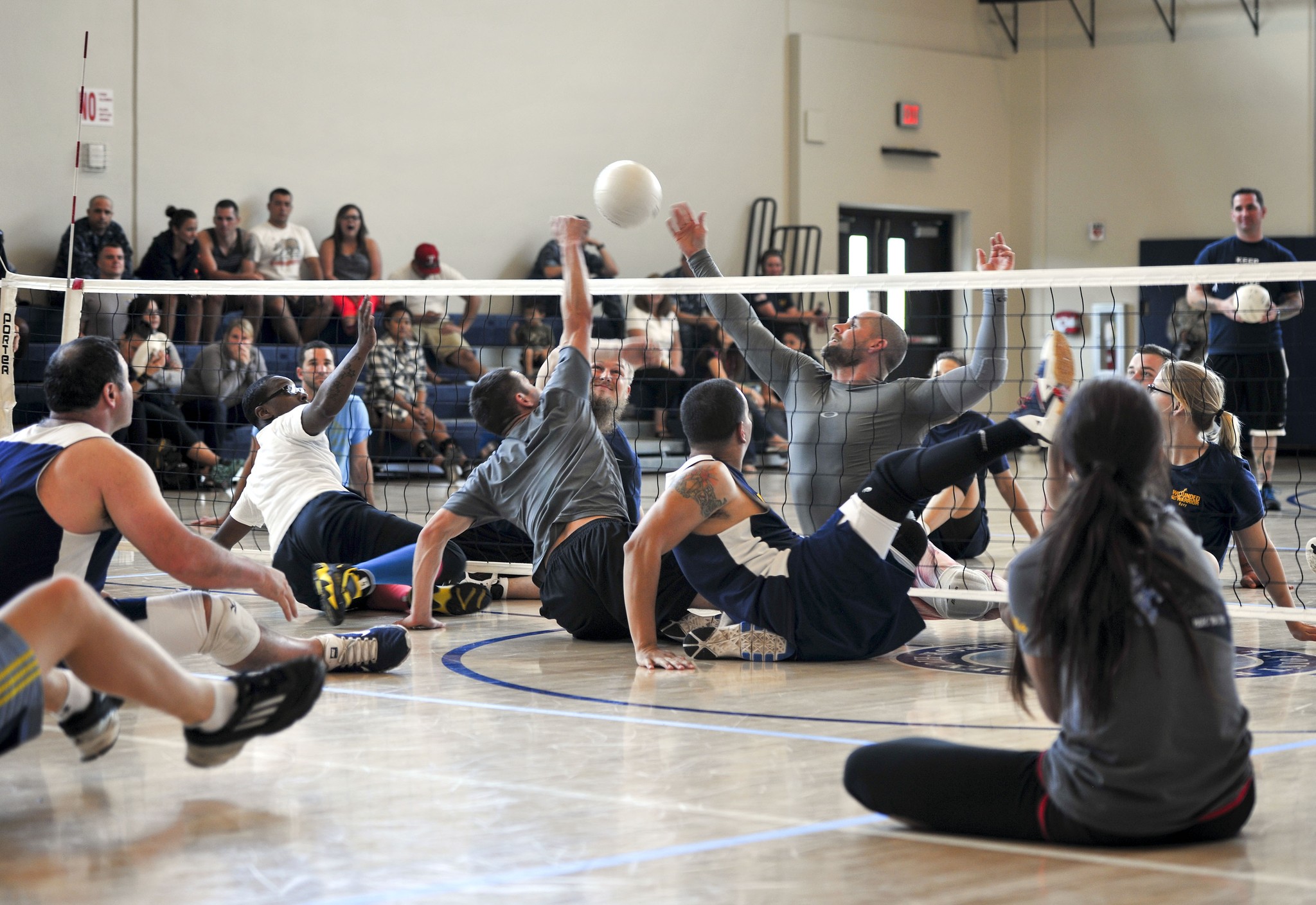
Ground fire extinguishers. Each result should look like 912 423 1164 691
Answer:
1106 348 1116 371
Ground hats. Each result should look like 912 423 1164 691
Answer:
413 241 442 276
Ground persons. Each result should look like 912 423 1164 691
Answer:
0 186 1316 670
0 576 328 767
839 373 1259 848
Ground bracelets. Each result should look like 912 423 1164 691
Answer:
597 243 605 249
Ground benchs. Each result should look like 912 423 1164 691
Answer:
9 305 790 476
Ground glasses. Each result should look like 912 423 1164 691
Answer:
258 384 307 405
1146 383 1174 399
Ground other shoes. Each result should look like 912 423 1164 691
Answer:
209 456 244 487
657 607 722 641
682 621 799 663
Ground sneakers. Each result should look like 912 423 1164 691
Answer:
441 571 504 601
1258 482 1282 511
406 582 494 616
1011 328 1076 448
58 688 127 762
314 625 412 676
311 560 376 626
183 653 326 769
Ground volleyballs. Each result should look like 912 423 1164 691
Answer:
594 160 662 229
1233 284 1272 323
